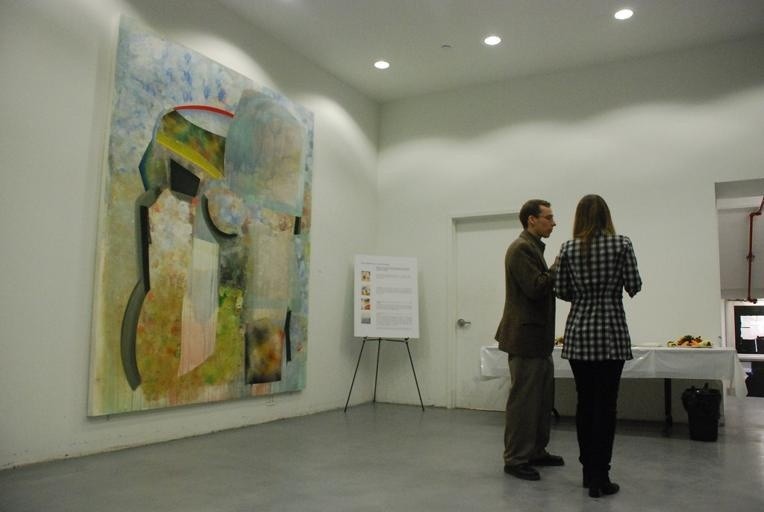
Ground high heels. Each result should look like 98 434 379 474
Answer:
589 478 619 497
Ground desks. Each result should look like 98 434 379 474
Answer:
481 344 749 439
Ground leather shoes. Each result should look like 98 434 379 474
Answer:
530 454 564 467
504 462 540 481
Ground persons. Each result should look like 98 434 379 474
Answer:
551 195 643 496
494 199 564 481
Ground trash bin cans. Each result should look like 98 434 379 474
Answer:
681 383 720 442
